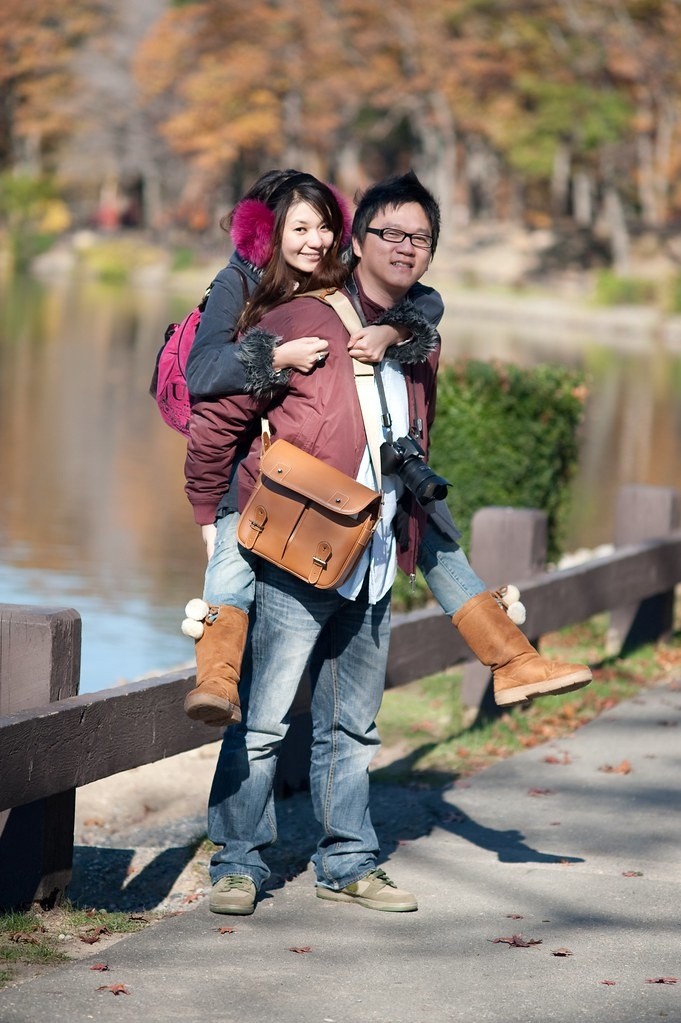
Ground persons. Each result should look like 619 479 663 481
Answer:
182 169 593 726
183 169 442 915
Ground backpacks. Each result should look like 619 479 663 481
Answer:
150 267 250 439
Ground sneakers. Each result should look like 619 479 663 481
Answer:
317 868 418 911
209 873 257 913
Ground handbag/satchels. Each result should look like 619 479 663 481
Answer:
233 438 382 588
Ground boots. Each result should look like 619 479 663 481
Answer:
451 584 593 706
182 598 249 727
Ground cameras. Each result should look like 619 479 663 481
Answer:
380 434 453 507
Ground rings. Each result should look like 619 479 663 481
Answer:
317 353 325 360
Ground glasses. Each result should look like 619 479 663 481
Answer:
366 228 435 248
